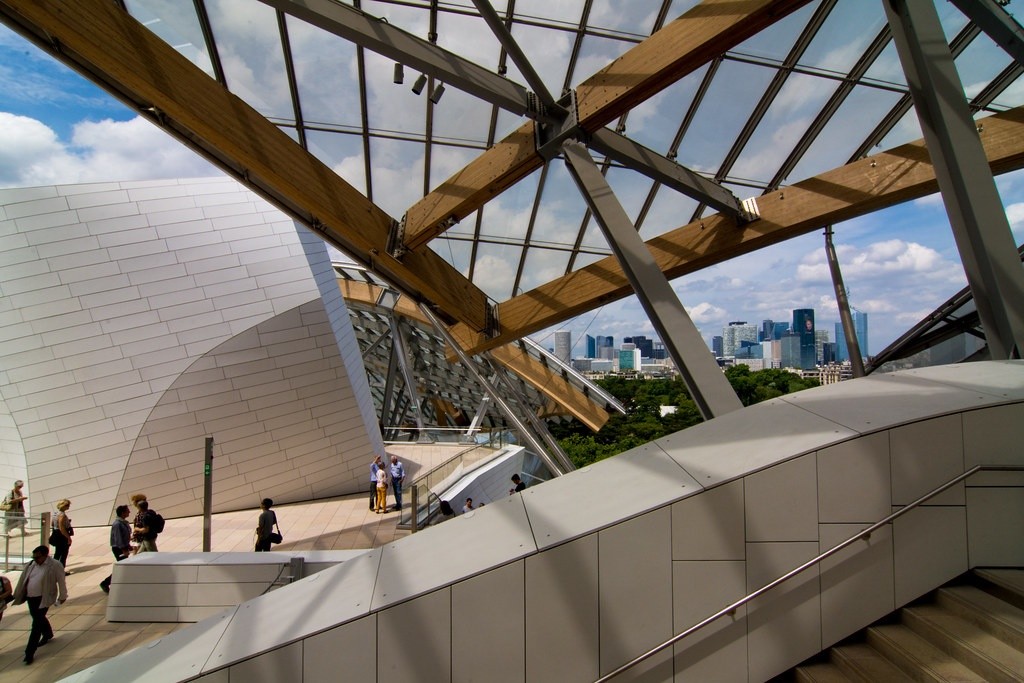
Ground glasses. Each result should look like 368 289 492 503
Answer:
31 554 43 560
16 485 22 488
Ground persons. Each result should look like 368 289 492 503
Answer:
478 503 484 507
369 455 381 512
389 455 406 511
99 505 132 593
51 499 73 576
0 575 12 622
463 498 475 513
11 545 68 662
131 493 158 556
4 480 28 538
375 462 388 514
430 501 456 525
254 498 277 553
511 473 526 493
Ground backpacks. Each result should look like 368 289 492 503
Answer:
147 509 165 533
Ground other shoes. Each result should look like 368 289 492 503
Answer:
383 510 389 513
392 505 400 510
370 509 375 512
38 636 51 647
25 655 35 665
376 512 379 514
65 572 70 576
379 506 382 509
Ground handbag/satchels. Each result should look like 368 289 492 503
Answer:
0 490 14 510
0 577 15 610
48 529 64 547
100 581 110 593
271 533 283 544
